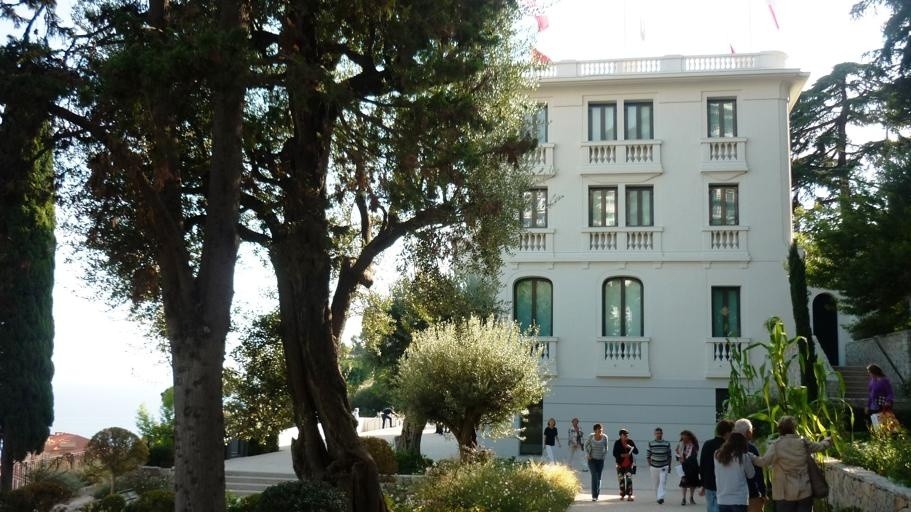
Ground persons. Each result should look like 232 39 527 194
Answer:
863 363 894 432
537 414 834 512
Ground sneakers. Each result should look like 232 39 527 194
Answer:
681 498 686 505
690 498 696 504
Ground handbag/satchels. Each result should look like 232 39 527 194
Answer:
806 455 829 498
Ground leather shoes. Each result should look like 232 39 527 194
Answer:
657 499 664 504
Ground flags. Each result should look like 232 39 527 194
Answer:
520 0 551 33
